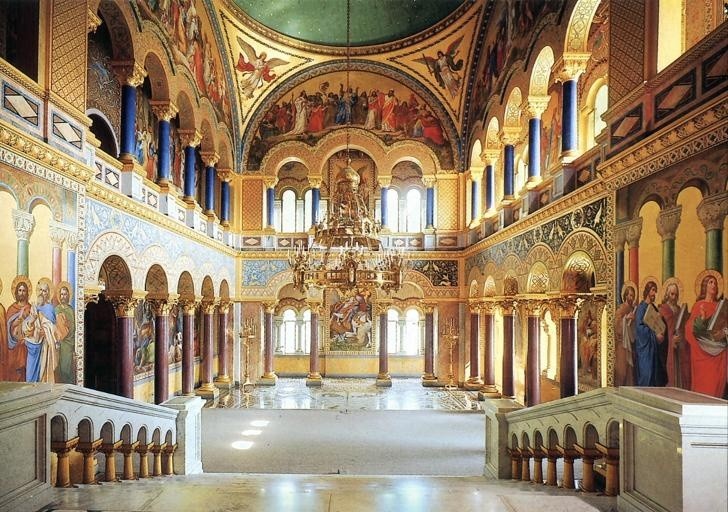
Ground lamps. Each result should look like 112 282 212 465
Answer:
291 0 405 295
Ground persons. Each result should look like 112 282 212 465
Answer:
0 281 8 383
21 283 57 383
615 286 635 385
685 275 728 398
6 281 32 382
660 283 690 389
53 286 75 383
330 296 371 348
134 308 199 373
634 281 668 385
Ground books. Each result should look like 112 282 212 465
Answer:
708 297 728 341
676 302 688 350
642 303 666 337
626 305 638 326
